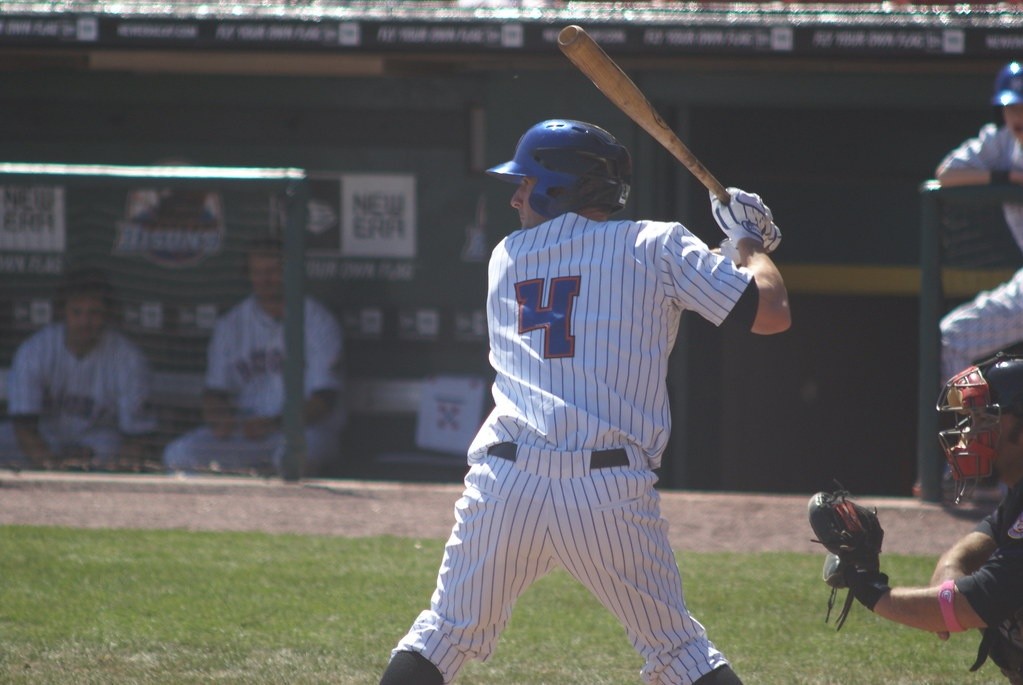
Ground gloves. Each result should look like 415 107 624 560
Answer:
719 221 782 266
708 187 772 248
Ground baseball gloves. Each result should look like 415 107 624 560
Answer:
807 475 893 632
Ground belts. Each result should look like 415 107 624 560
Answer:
487 442 630 468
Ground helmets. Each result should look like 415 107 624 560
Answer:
982 358 1023 415
485 119 632 219
991 62 1023 106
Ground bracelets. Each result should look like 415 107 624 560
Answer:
938 581 967 633
990 168 1010 183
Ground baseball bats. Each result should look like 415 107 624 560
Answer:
556 23 731 206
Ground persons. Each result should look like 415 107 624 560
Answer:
935 60 1023 372
159 237 350 478
808 347 1023 685
378 118 792 685
0 266 151 473
